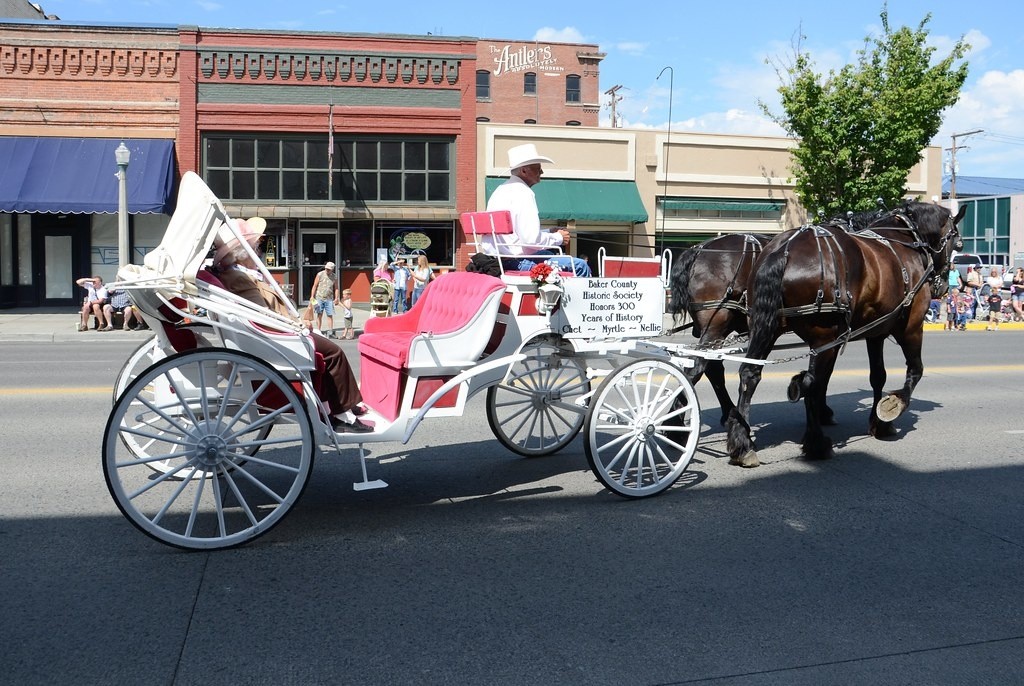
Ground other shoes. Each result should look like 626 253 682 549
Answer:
97 324 105 331
328 330 337 339
994 327 999 331
987 326 992 331
1014 315 1021 322
103 325 113 331
338 335 345 339
78 325 88 332
134 323 144 331
346 336 354 340
123 323 130 331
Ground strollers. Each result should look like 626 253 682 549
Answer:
975 283 1014 324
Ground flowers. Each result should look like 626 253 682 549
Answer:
530 263 554 287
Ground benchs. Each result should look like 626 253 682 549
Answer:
359 272 506 370
198 270 316 372
79 297 124 326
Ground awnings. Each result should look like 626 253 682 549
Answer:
484 178 648 225
658 199 786 211
0 137 174 215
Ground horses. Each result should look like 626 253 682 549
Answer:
660 200 968 467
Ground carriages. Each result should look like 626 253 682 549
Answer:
101 170 969 552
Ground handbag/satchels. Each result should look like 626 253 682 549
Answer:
100 303 105 311
303 303 314 321
1011 285 1015 294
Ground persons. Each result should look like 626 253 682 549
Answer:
930 264 1024 331
373 255 435 315
482 144 591 276
310 262 354 340
76 277 152 331
214 217 373 433
581 255 588 261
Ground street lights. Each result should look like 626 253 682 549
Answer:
114 140 131 268
656 66 674 259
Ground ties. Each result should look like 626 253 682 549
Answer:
978 273 981 285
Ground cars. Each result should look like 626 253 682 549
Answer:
1002 266 1013 286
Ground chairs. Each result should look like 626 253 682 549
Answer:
460 211 576 284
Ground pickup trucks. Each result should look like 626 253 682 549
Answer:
951 253 985 285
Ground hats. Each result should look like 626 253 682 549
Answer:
213 216 266 265
325 262 335 271
973 263 982 269
498 144 555 178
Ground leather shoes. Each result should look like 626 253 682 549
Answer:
328 413 374 433
353 405 365 415
